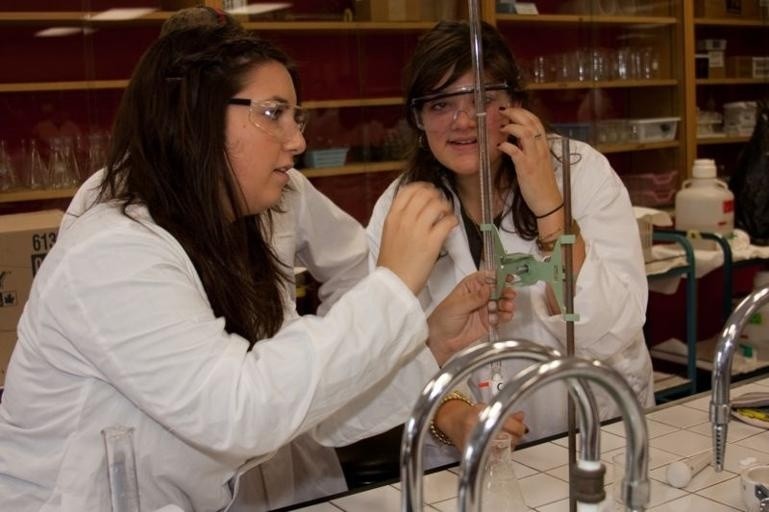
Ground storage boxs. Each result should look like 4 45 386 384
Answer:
549 121 592 144
0 209 79 330
0 330 19 390
693 0 762 20
693 36 769 82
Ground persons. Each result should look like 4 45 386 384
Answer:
2 23 515 512
365 18 656 474
53 5 369 511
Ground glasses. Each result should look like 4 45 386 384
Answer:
228 99 311 142
409 82 509 132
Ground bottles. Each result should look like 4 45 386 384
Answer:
0 132 110 194
475 429 528 512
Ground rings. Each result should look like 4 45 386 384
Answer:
535 134 541 139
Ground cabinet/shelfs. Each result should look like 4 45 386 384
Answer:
483 0 769 216
0 0 483 220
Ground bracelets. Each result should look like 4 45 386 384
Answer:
427 391 475 447
532 198 564 220
532 219 582 252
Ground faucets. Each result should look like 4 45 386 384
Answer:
707 286 769 474
397 336 608 512
456 356 652 512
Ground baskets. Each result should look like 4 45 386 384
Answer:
549 123 605 142
620 170 682 190
636 215 654 263
727 56 768 78
628 190 676 206
300 148 350 168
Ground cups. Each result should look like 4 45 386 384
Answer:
611 450 653 506
739 465 768 512
526 46 659 84
